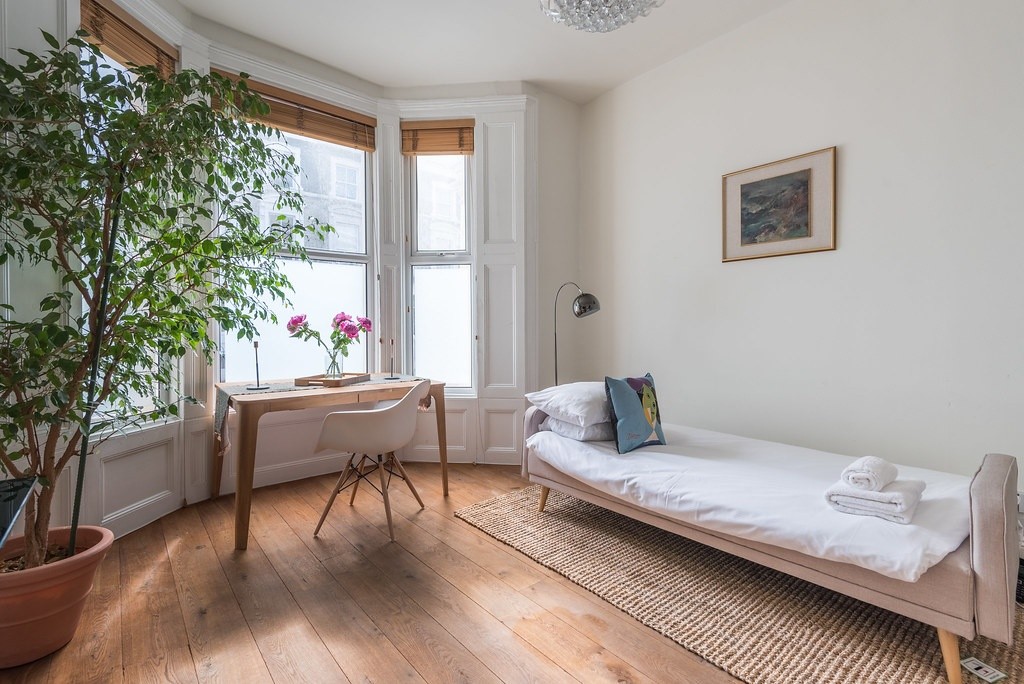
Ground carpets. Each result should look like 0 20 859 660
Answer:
453 483 1024 684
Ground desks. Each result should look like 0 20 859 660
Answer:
209 373 449 550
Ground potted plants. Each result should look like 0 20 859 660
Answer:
0 27 340 669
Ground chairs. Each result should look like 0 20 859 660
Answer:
312 380 431 543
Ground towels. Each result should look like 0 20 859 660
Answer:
841 456 897 492
824 480 926 524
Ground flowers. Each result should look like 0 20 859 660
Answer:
286 312 372 357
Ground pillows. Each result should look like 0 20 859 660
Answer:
604 372 666 454
537 416 615 442
525 382 611 428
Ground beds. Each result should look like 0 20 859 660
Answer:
520 407 1019 684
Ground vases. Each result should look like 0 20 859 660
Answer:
322 349 343 379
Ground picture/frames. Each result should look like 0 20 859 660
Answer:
722 147 837 263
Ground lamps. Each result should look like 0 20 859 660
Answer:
554 282 600 386
536 0 665 34
